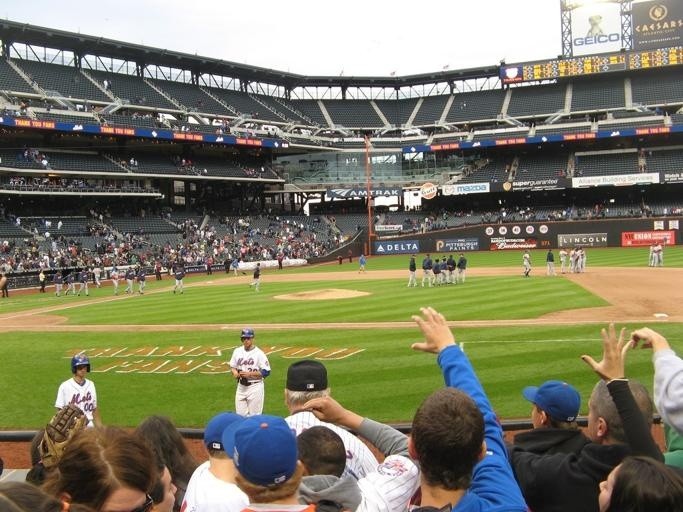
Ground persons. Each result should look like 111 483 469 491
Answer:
504 161 518 179
649 242 664 267
522 250 531 276
546 245 586 276
407 253 467 287
0 148 353 296
664 236 667 248
382 203 683 231
559 163 583 176
358 255 366 275
638 149 646 174
0 307 683 512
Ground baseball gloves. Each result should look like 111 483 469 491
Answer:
38 401 90 468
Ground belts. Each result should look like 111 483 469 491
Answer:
237 379 258 386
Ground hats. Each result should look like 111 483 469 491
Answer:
285 360 328 392
203 413 244 450
522 379 581 421
222 415 297 485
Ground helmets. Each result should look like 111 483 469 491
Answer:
240 328 254 338
71 353 90 373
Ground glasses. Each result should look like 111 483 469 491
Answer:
129 495 153 512
